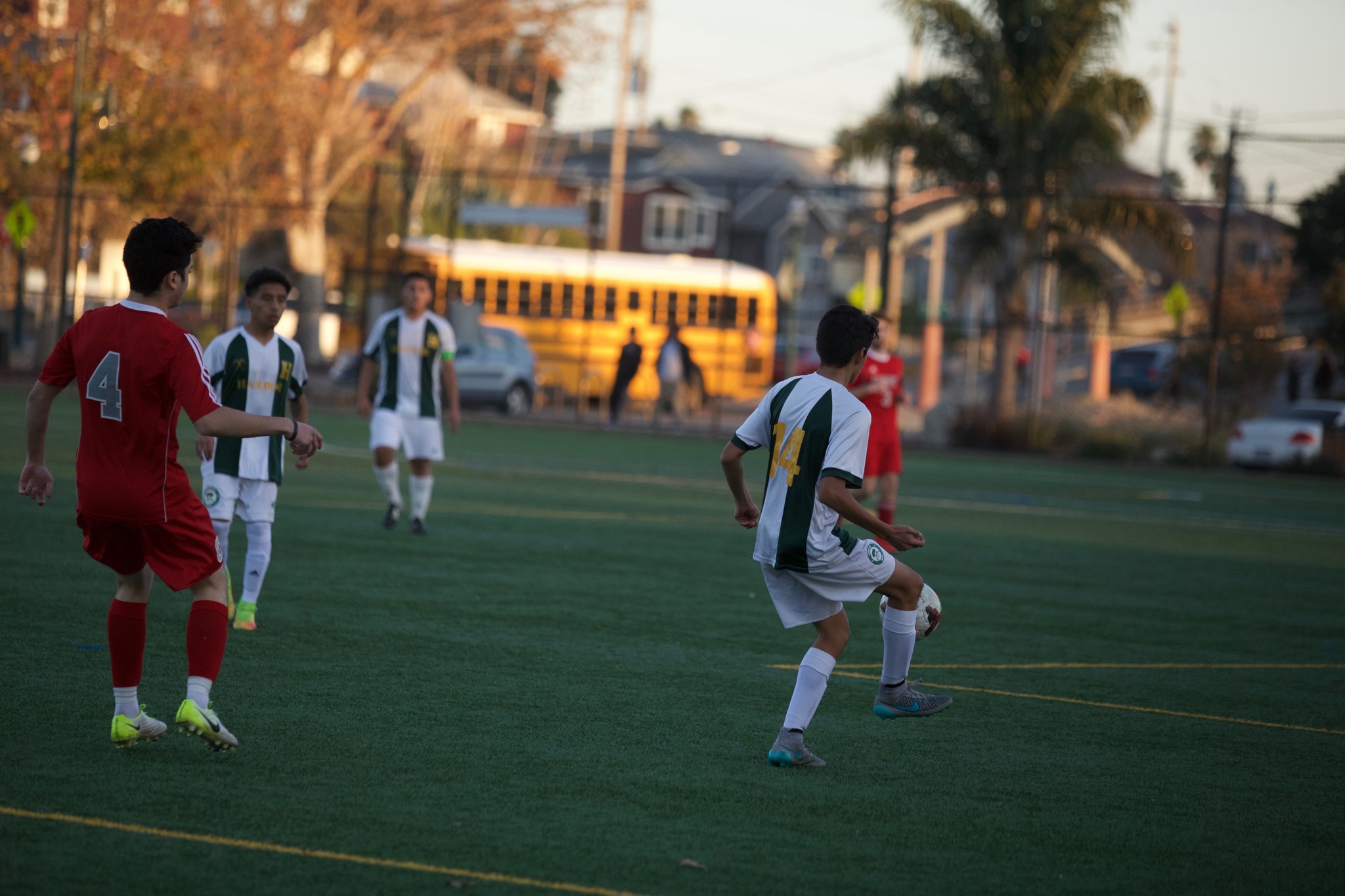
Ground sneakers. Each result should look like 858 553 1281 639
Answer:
873 535 901 555
225 572 235 621
110 704 167 748
873 683 953 721
408 516 427 536
767 727 826 768
233 599 258 630
176 699 239 752
384 503 401 529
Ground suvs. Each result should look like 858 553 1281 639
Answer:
328 325 536 421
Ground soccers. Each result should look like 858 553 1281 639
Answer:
878 582 942 642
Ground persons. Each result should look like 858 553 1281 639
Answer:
17 216 324 756
652 314 697 425
822 313 912 557
717 302 955 772
185 267 324 633
356 272 463 538
605 325 644 432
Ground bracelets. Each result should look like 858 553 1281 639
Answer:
284 417 300 442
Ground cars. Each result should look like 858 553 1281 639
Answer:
1228 399 1344 474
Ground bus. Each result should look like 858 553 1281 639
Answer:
367 235 777 407
